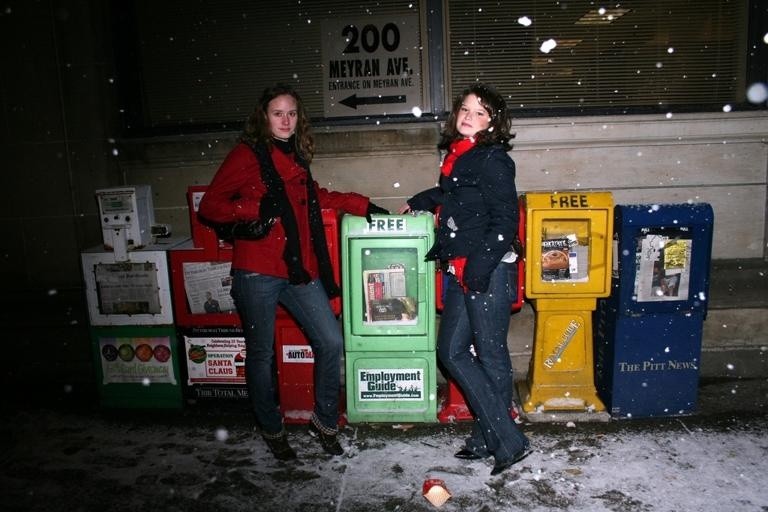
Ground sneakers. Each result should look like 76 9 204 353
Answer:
308 420 344 455
264 434 297 461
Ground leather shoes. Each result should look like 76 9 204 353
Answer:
454 449 481 459
491 450 533 475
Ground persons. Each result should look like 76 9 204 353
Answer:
395 82 537 475
197 82 390 462
204 291 221 313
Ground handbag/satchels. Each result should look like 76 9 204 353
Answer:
214 215 273 240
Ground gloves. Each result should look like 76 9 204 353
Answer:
365 202 390 223
259 193 289 217
